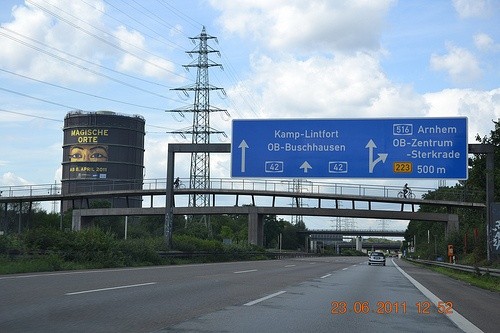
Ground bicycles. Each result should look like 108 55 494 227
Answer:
397 189 416 200
173 181 186 190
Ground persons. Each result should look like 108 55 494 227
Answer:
403 183 409 198
175 177 180 187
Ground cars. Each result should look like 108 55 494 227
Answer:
388 252 398 258
368 251 386 266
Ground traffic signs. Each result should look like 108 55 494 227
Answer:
230 116 469 180
